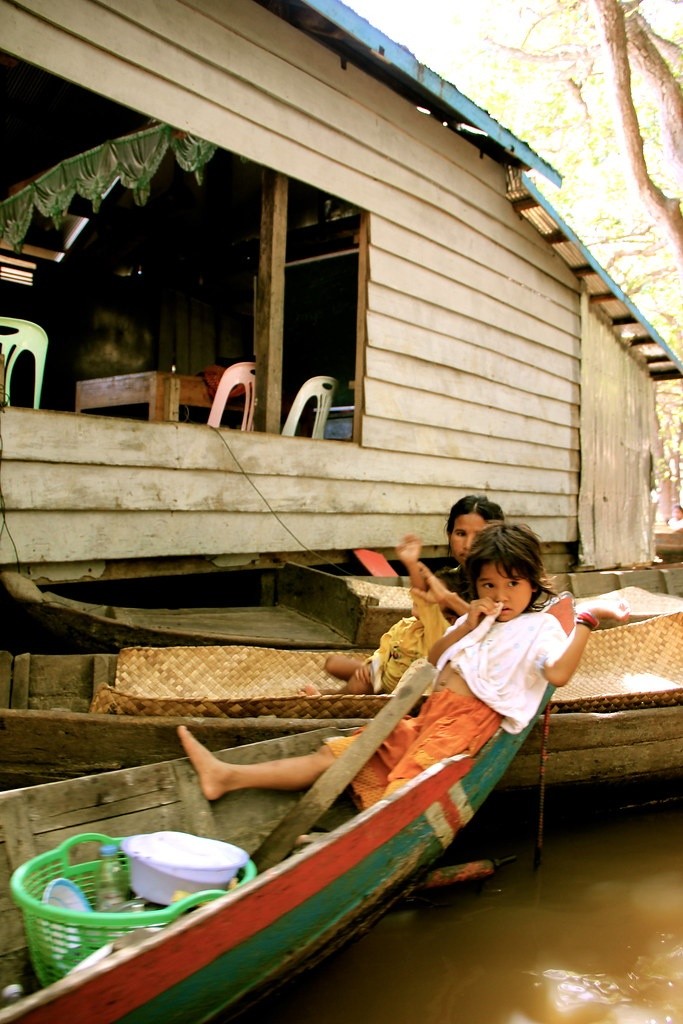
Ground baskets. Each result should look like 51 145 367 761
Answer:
8 831 257 989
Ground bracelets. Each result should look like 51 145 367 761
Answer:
576 610 600 630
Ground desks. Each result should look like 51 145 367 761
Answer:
74 369 244 424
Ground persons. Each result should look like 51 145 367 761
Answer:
326 495 506 694
176 518 630 801
667 505 683 532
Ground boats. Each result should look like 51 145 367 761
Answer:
1 589 574 1023
0 650 682 818
4 559 683 653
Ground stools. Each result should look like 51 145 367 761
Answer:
206 361 256 432
0 316 47 409
281 375 337 439
